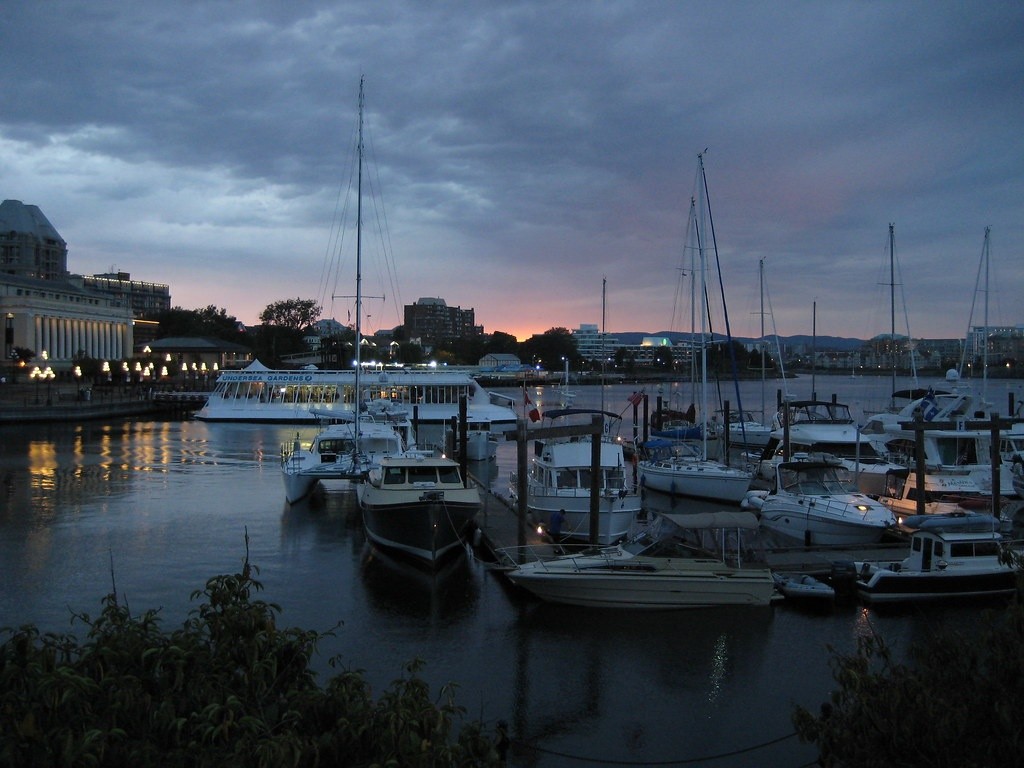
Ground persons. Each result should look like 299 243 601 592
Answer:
221 382 340 404
550 509 566 554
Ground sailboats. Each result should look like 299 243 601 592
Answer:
620 148 791 507
860 221 1024 533
278 71 436 505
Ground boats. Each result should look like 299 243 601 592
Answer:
193 358 519 424
444 413 500 461
737 450 898 547
359 457 482 585
853 514 1024 604
509 408 642 546
494 511 775 607
769 301 872 459
772 571 835 611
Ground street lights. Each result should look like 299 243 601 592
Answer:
27 349 57 404
561 356 569 384
121 361 219 394
73 365 82 402
101 360 110 396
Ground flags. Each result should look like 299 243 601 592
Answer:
524 386 540 423
920 387 942 422
